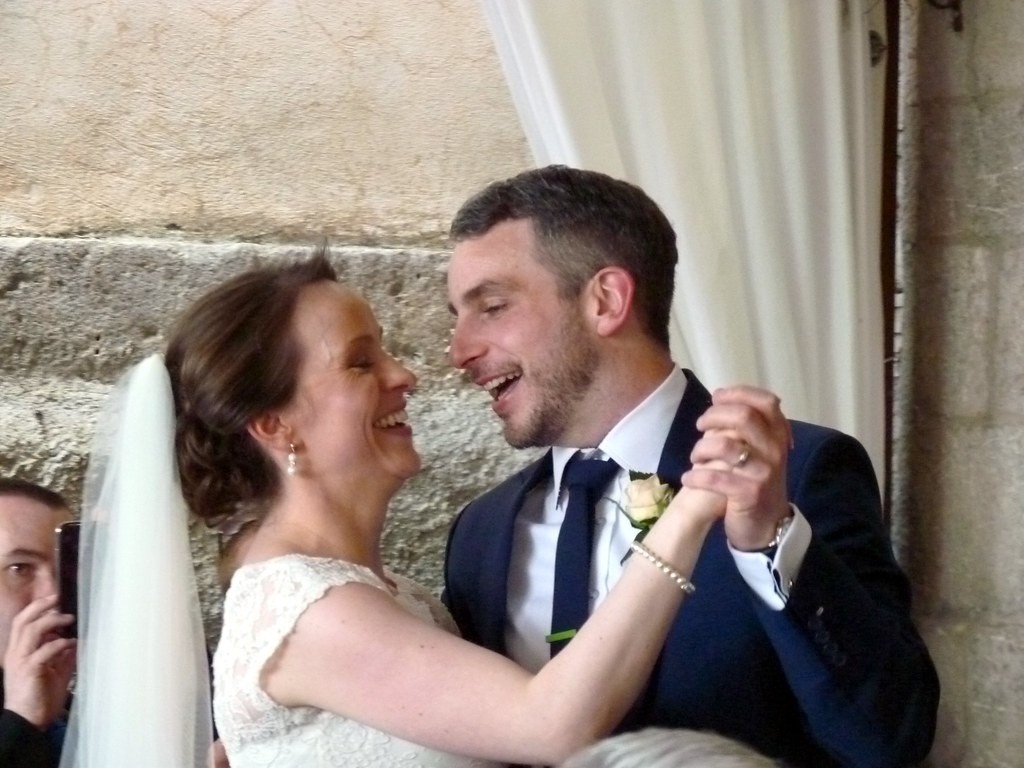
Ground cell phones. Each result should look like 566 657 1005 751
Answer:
53 520 82 639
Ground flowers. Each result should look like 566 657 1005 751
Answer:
600 472 674 565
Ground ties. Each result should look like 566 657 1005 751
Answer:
549 458 619 659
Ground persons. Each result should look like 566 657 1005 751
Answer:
0 477 230 768
60 254 779 768
443 164 940 768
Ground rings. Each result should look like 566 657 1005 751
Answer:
734 443 751 467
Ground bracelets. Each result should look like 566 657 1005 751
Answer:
631 541 694 595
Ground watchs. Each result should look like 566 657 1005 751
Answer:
755 516 793 559
